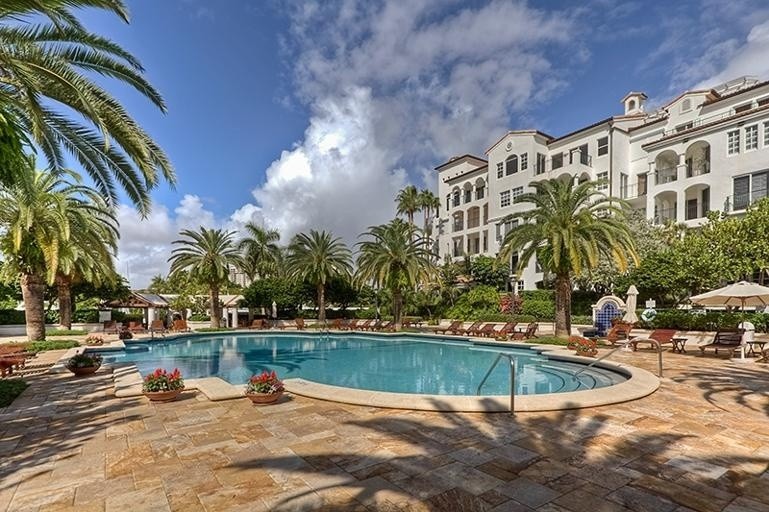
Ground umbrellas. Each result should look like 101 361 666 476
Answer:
270 301 279 326
622 284 642 346
690 280 769 357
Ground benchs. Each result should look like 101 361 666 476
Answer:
275 319 306 330
248 320 263 329
403 317 426 328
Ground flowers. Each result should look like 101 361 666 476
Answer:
568 335 595 350
143 367 184 392
63 353 102 368
119 331 132 338
242 367 284 394
87 336 103 344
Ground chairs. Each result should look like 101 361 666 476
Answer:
698 322 755 359
333 318 393 332
606 324 679 350
102 318 191 334
434 320 539 340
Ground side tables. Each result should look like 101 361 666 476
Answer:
670 338 688 354
746 340 768 358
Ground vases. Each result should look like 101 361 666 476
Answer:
576 350 598 357
245 390 284 405
68 365 101 376
141 387 184 403
87 343 102 346
